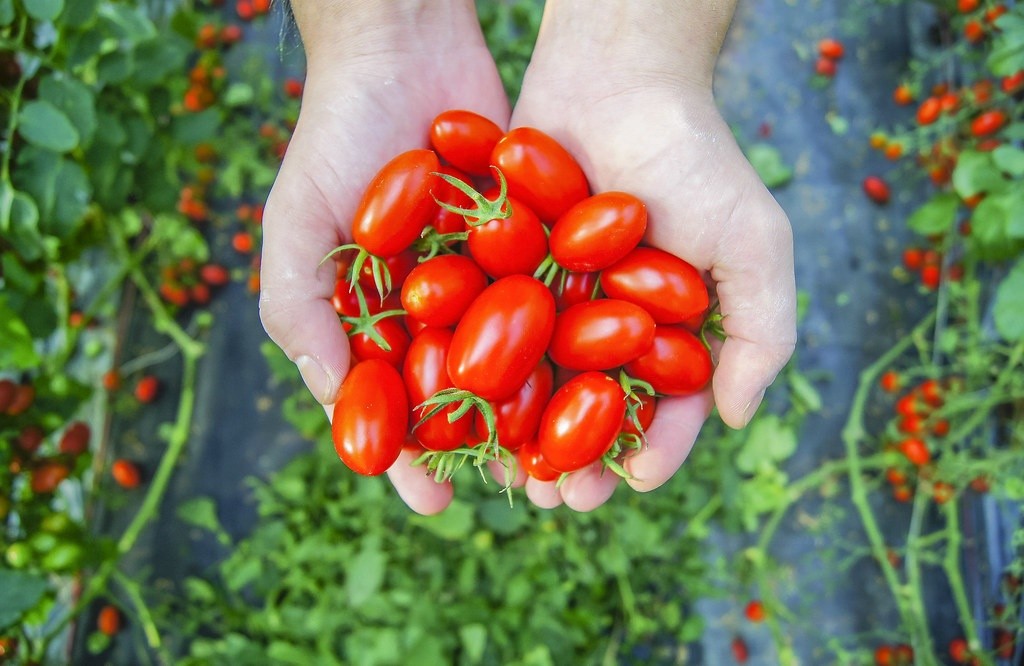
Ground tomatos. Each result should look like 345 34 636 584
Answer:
733 1 1024 666
331 112 729 510
0 1 307 658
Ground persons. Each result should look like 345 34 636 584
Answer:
258 0 799 518
